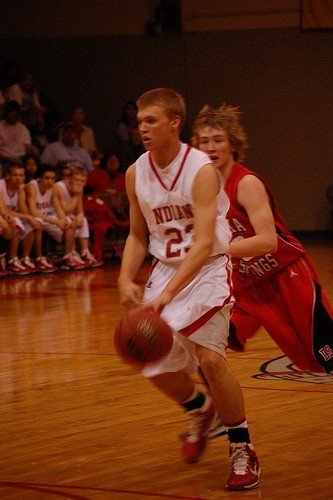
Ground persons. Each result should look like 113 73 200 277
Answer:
0 70 146 277
178 104 333 441
118 88 261 489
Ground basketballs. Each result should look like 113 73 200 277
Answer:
114 310 174 372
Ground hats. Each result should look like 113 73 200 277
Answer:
2 101 19 109
53 121 86 131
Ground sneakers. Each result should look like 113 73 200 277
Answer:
225 443 260 491
61 253 87 270
34 256 58 272
72 251 93 268
0 257 13 277
21 256 40 273
178 411 227 442
8 256 31 275
81 250 104 267
178 392 217 465
